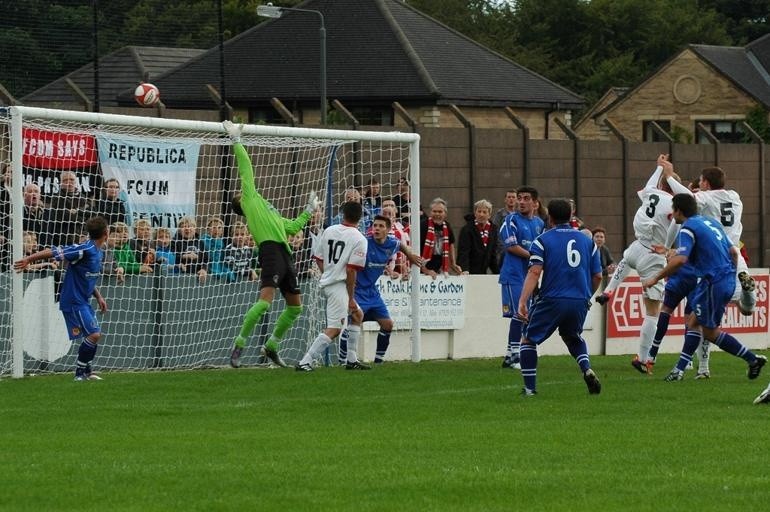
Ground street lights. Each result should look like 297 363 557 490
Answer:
256 3 329 127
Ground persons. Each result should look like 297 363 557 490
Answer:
688 166 756 316
1 158 462 286
293 199 375 374
640 190 769 383
456 190 618 276
655 158 760 381
495 184 548 372
221 117 322 371
336 214 424 367
13 215 111 383
515 195 604 398
593 152 683 376
752 382 770 405
647 177 704 372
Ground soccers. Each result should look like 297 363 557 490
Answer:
135 81 163 108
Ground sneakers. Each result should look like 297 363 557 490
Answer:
738 271 756 292
85 374 103 382
685 361 694 370
752 384 769 405
510 357 520 369
595 291 612 305
665 369 684 383
346 360 372 370
632 357 649 374
694 371 710 380
584 368 601 395
230 343 244 368
747 355 768 380
519 388 536 396
261 345 287 367
338 360 346 367
74 373 89 381
502 359 511 368
295 361 314 372
